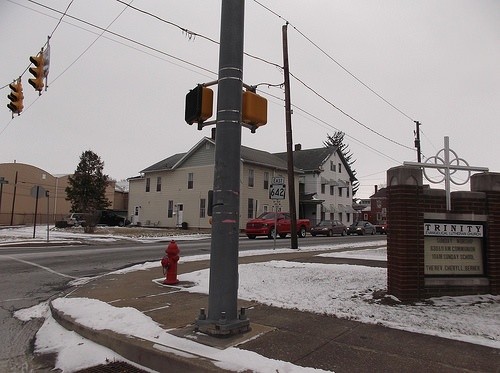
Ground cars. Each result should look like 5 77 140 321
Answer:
347 220 376 235
67 212 89 226
376 219 388 234
310 219 347 236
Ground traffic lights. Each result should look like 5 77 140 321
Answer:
28 52 44 91
185 86 213 125
7 83 24 112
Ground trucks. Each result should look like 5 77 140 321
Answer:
96 208 131 226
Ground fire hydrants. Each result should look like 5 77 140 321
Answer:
161 239 181 284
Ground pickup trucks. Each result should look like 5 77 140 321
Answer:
245 211 310 239
